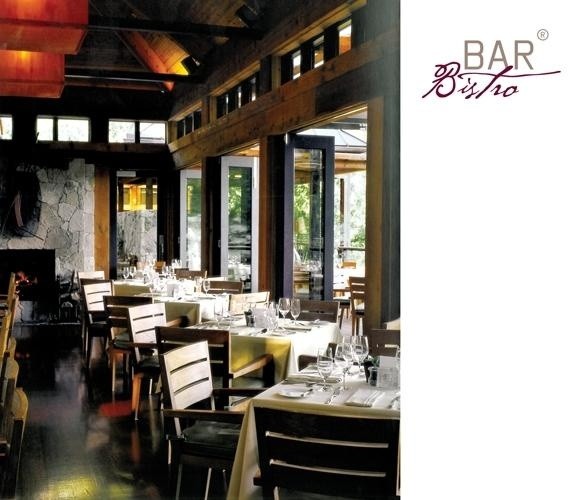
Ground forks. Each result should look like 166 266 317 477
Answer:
325 388 340 406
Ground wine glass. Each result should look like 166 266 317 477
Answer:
277 298 301 326
317 336 370 393
123 258 183 285
193 276 210 296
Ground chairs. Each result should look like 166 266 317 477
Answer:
3 270 29 499
62 259 399 497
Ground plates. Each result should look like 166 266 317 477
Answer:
276 387 311 398
197 294 218 300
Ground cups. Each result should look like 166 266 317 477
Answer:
252 307 278 330
367 365 398 389
